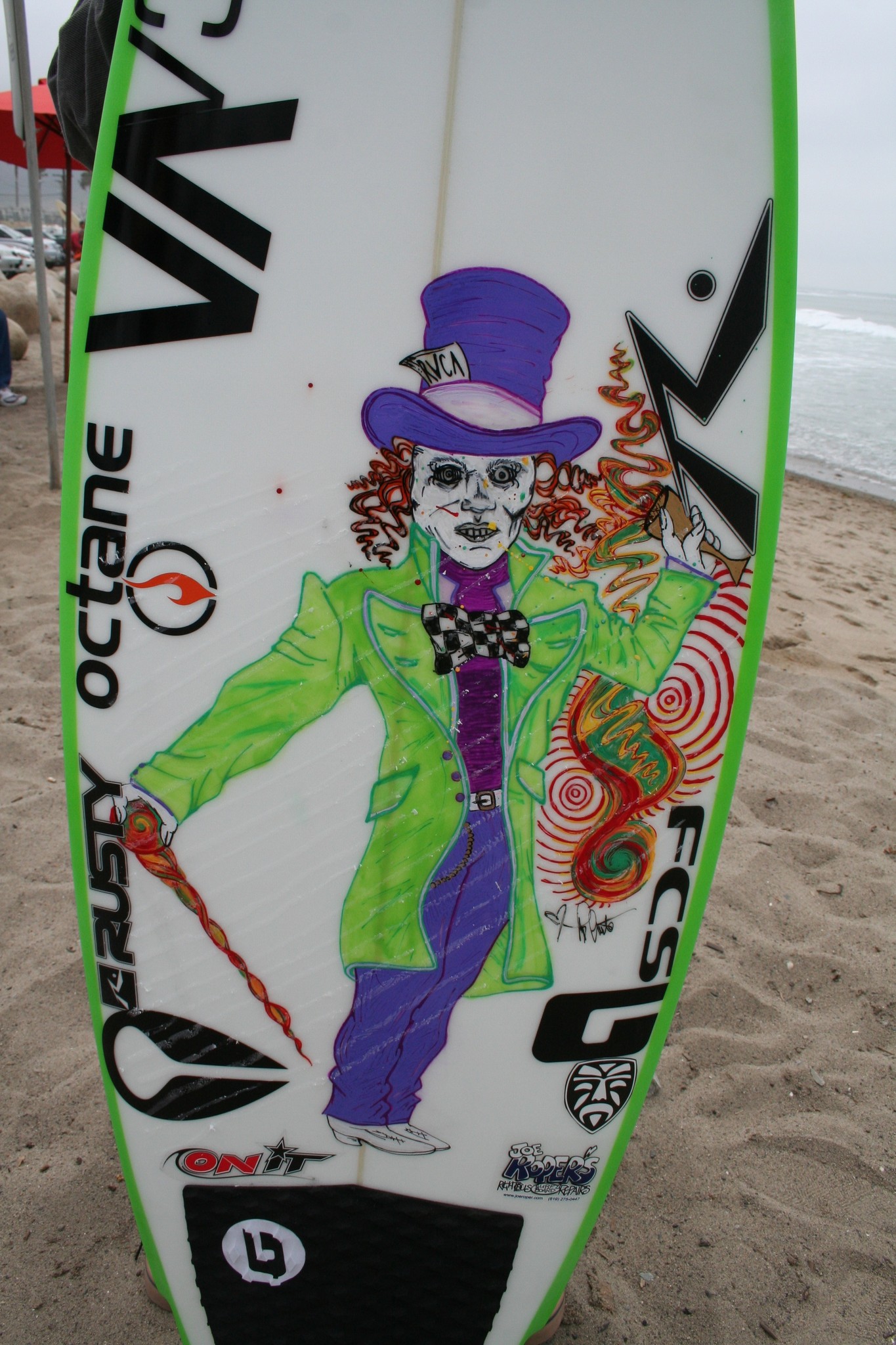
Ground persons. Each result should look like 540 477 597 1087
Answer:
77 219 86 244
48 0 123 171
0 309 28 408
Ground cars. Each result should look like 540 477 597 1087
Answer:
0 223 66 273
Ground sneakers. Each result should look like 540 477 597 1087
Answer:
0 387 28 406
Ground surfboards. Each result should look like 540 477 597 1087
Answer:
61 2 796 1345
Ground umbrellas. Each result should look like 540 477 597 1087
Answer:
0 79 92 383
